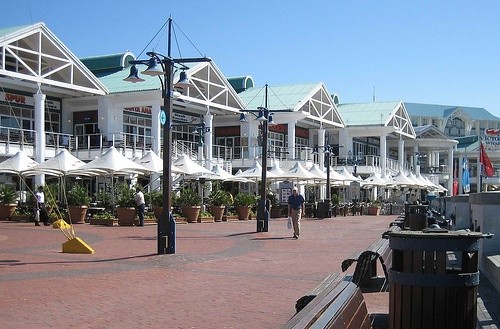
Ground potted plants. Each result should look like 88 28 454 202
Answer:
369 203 381 215
64 185 90 223
266 193 283 218
0 184 19 219
116 187 138 226
210 190 230 221
90 215 114 226
179 188 202 223
40 184 59 214
232 194 255 220
153 192 176 221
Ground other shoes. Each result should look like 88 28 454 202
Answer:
295 235 299 239
35 223 41 226
44 223 51 226
136 225 144 227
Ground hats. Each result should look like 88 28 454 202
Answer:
292 187 298 191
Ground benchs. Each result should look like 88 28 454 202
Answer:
281 213 404 329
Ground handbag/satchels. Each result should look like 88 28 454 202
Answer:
35 210 40 222
286 216 293 230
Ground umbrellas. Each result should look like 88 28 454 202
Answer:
0 147 446 204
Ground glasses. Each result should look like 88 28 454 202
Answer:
293 190 296 191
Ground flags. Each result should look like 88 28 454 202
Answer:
454 163 458 195
479 141 493 177
463 155 470 193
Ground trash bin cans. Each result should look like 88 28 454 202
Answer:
405 203 418 227
317 203 326 219
409 205 429 231
388 225 482 329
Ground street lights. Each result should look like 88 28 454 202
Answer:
238 83 293 234
123 18 212 254
310 131 344 219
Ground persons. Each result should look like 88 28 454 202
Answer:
33 186 50 226
134 187 145 226
287 187 305 239
